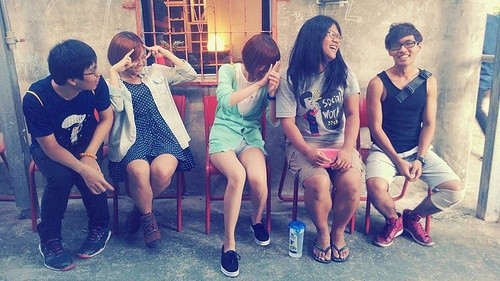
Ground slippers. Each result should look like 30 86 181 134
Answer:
313 232 349 264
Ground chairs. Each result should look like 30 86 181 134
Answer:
113 95 185 234
203 96 271 234
356 89 439 235
278 133 356 235
29 109 107 233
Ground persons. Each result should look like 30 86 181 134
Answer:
22 39 112 271
275 14 364 263
366 22 463 247
208 34 284 276
103 30 198 247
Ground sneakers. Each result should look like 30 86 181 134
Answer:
374 212 403 246
402 209 434 246
77 224 111 258
126 204 161 248
220 243 241 277
250 215 270 246
38 224 73 270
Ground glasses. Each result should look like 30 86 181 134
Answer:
326 30 343 40
390 40 418 50
84 67 97 75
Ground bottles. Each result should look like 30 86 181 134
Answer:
288 220 306 259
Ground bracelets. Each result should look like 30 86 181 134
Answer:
267 93 276 100
413 155 426 166
79 152 98 160
